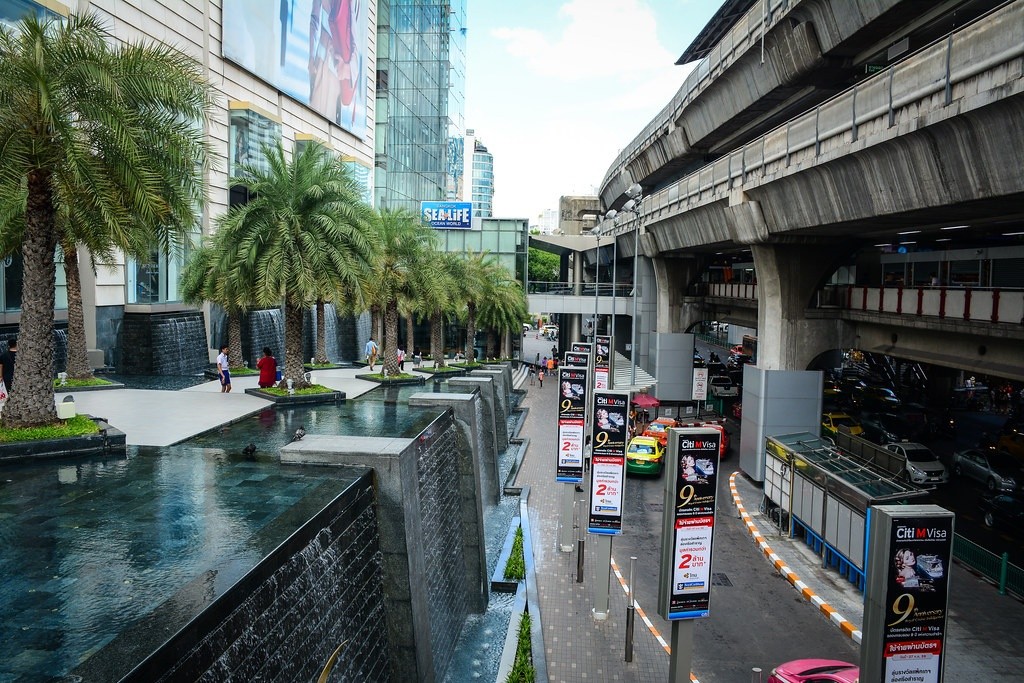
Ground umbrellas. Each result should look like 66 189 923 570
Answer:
630 393 660 425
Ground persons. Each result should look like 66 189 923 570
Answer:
530 364 536 386
561 379 574 399
541 357 547 375
412 345 422 368
0 338 18 395
675 416 682 421
396 344 406 372
308 0 361 129
596 409 613 429
538 369 545 388
365 337 379 371
545 353 559 376
628 409 650 433
256 347 276 388
681 454 700 482
551 345 557 359
535 353 539 370
895 548 922 587
216 346 231 393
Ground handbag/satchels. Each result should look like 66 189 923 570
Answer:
398 357 400 364
372 343 376 356
272 357 282 381
411 354 415 359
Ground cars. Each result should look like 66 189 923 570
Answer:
540 325 559 338
626 417 729 480
821 366 1024 536
768 659 860 683
711 321 728 333
694 344 754 375
523 323 533 331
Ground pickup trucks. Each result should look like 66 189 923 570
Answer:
707 374 739 397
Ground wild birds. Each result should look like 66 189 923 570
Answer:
242 443 256 456
291 425 305 442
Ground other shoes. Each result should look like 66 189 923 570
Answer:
370 366 373 371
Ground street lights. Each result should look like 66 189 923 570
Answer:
608 210 617 389
623 181 642 387
592 227 600 388
